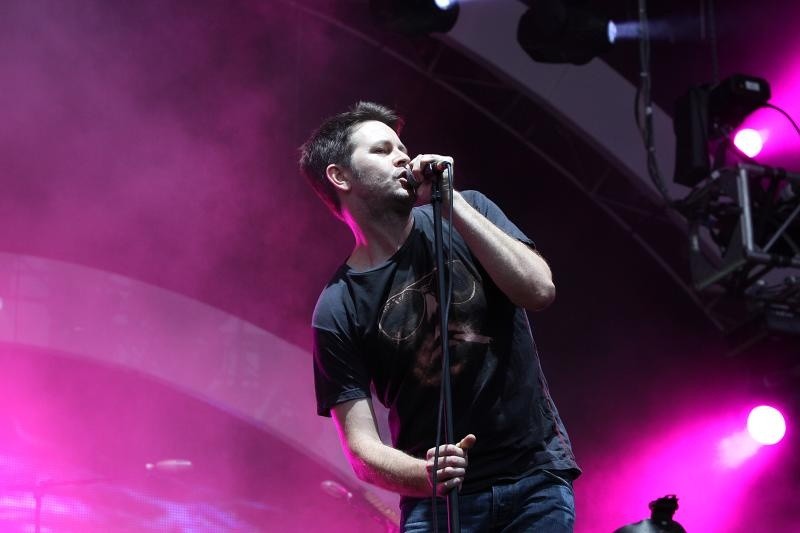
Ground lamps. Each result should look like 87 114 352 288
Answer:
392 0 770 139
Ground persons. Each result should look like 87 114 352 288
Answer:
296 102 581 533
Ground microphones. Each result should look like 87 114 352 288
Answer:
406 161 451 188
